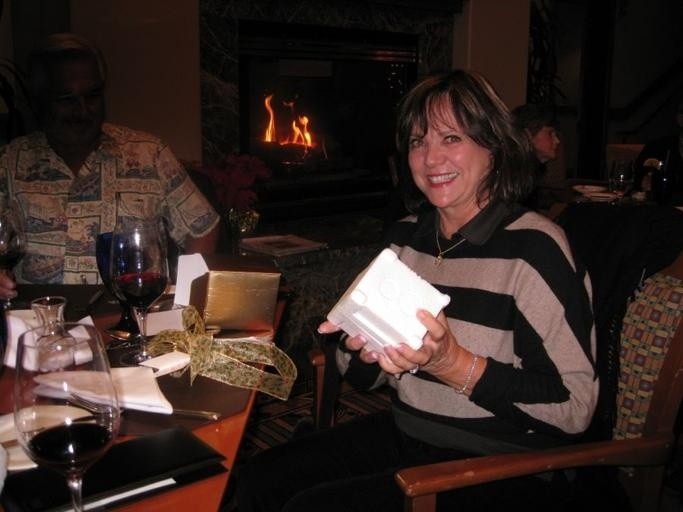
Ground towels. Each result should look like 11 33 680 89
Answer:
31 364 175 416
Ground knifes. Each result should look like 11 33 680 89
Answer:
1 410 99 448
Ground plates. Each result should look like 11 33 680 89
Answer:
572 184 607 194
0 400 97 476
585 192 617 202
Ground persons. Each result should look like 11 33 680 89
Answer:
510 105 589 222
220 70 599 512
0 35 220 299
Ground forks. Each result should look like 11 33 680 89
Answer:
66 389 223 426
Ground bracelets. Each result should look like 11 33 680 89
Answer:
456 353 479 395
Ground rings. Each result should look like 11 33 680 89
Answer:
407 363 418 374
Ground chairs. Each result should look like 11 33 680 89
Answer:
303 264 682 511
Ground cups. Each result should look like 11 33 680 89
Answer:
93 226 172 368
609 176 627 201
9 322 121 511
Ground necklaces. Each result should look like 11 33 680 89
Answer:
434 227 468 267
